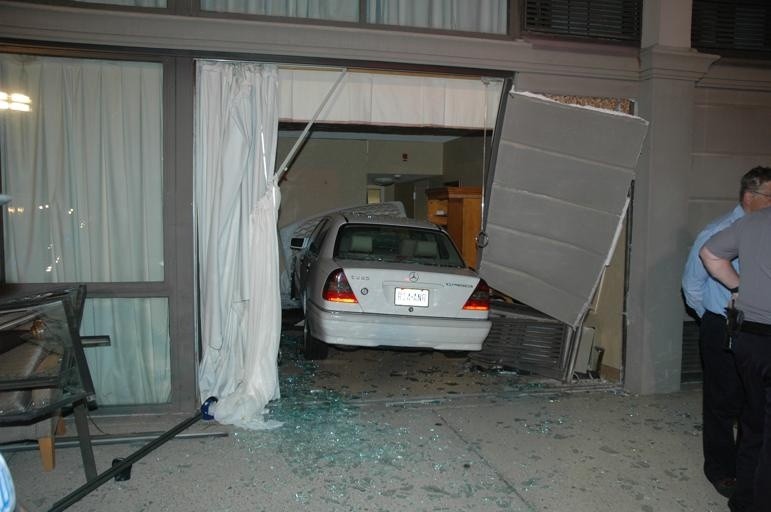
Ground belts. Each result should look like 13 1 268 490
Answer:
740 319 771 339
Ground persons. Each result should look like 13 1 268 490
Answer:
679 165 770 498
697 207 770 512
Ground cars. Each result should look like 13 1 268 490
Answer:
290 214 492 359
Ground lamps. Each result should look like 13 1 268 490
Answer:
1 53 33 122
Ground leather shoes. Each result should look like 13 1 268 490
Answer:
703 462 739 498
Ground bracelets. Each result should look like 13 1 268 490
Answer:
729 286 738 294
